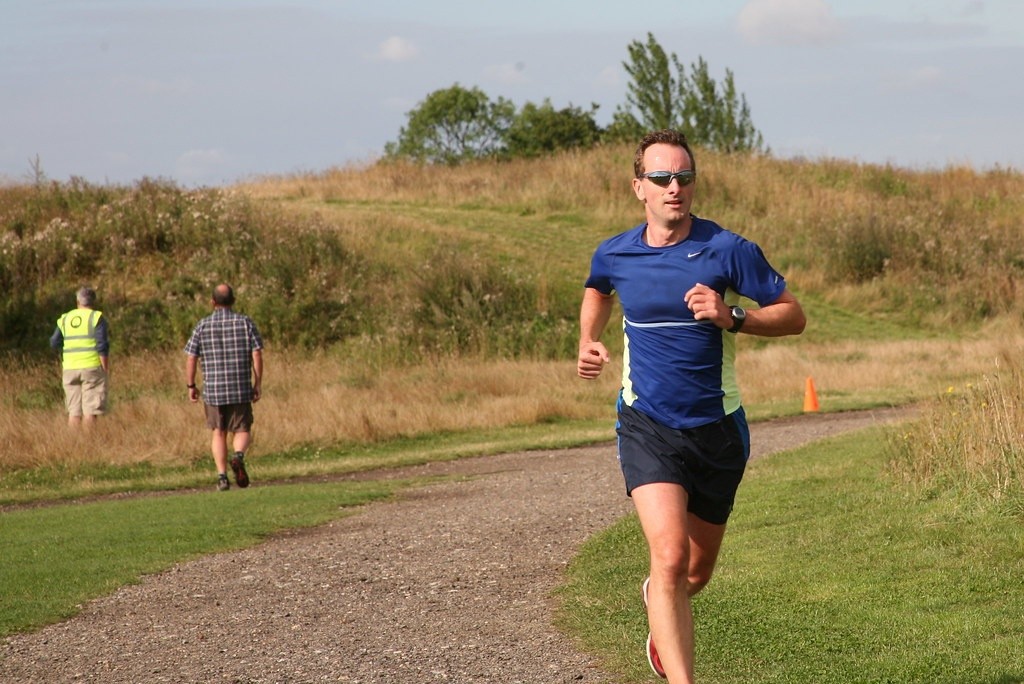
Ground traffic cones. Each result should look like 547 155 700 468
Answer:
803 377 820 413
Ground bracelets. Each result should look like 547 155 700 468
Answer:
186 384 195 388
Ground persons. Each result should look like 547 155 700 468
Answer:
577 131 807 683
50 286 110 425
183 284 264 492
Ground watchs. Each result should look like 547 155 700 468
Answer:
727 305 746 333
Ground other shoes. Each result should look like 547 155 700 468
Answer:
216 474 231 491
230 457 250 488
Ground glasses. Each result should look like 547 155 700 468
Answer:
641 170 695 186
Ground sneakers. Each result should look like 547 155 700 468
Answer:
642 576 667 680
